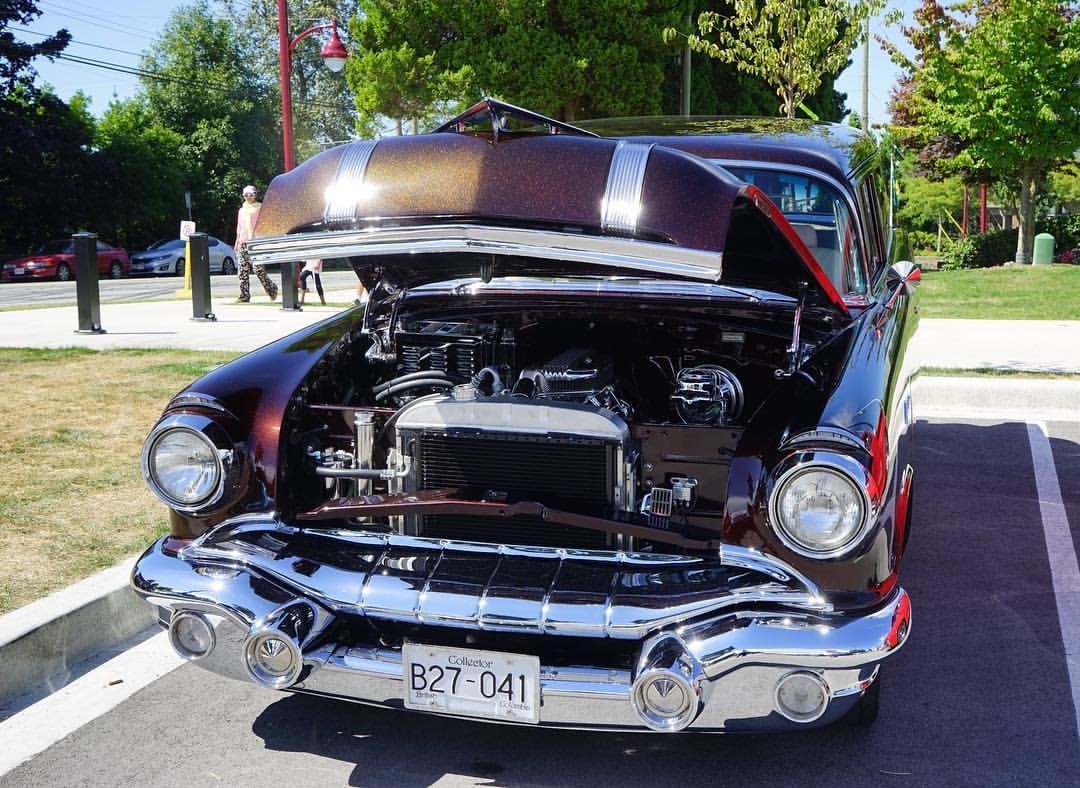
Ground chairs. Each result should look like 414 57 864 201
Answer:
793 225 842 292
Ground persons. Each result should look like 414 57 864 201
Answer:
298 259 326 307
350 279 364 308
233 185 278 304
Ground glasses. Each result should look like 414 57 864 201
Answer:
245 194 254 198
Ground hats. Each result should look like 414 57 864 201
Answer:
242 185 257 195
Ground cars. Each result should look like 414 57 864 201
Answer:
130 233 240 275
1 237 129 281
128 98 923 738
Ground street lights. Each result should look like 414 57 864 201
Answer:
275 0 349 287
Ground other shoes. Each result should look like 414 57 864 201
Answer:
271 292 277 300
235 299 243 303
